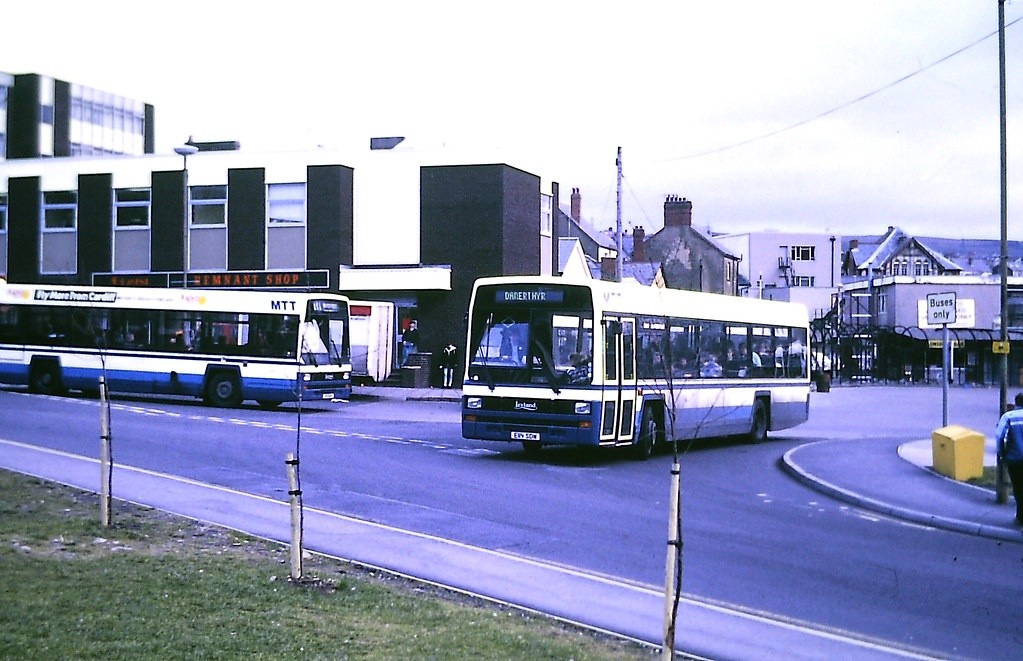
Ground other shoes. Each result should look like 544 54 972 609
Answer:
400 364 403 367
441 386 452 389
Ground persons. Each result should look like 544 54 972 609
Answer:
39 310 298 357
400 321 418 366
553 324 807 385
437 340 459 389
994 391 1023 528
498 328 528 364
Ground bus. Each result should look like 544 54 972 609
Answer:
460 276 811 460
1 282 353 410
